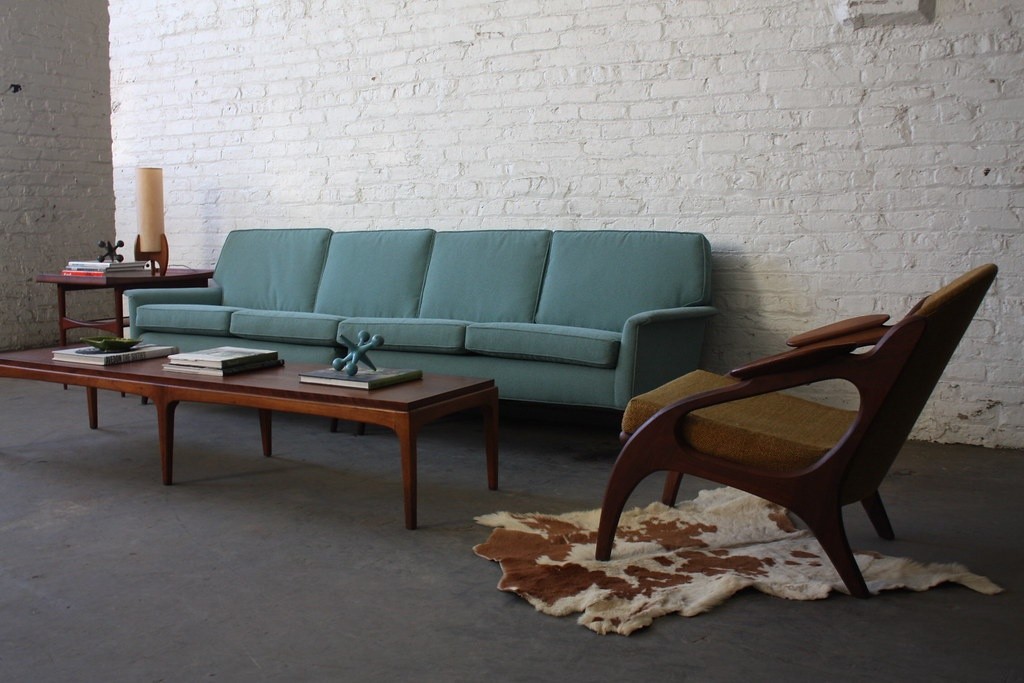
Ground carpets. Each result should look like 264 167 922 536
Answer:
471 487 1004 636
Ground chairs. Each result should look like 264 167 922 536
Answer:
595 263 1000 600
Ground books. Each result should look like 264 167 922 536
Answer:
52 343 179 365
298 367 423 390
61 260 147 276
161 346 285 377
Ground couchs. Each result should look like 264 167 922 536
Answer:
123 228 718 437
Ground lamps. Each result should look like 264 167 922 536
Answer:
134 167 169 276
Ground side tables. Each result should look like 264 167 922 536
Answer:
36 268 215 398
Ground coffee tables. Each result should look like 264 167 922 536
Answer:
0 343 498 531
